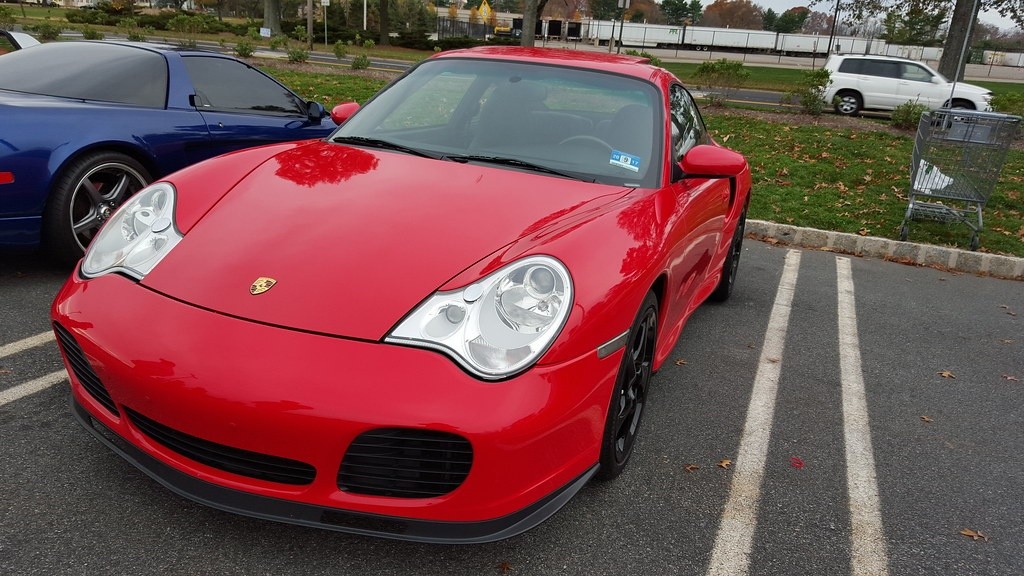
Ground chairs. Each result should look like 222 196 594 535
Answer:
602 103 651 160
470 85 535 147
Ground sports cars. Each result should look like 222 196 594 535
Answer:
48 44 755 548
0 41 341 274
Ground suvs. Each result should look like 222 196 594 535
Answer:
809 51 994 129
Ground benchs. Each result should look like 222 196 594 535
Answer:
534 109 619 142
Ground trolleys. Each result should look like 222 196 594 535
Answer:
897 106 1024 252
